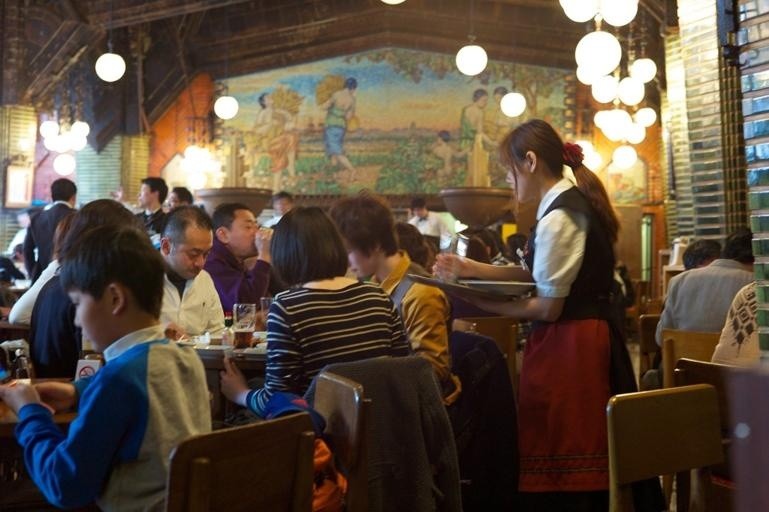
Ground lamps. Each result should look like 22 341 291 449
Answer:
93 0 127 84
558 0 658 176
380 1 404 5
500 1 527 117
210 2 240 121
456 1 489 77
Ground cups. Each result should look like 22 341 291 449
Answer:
185 328 211 348
232 303 256 348
252 331 268 349
260 296 274 328
433 232 469 283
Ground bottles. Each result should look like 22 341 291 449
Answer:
222 325 233 346
85 353 103 370
11 346 31 384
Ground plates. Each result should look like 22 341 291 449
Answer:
191 344 231 352
462 278 535 297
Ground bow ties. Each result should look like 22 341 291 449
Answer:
419 217 427 222
529 220 541 249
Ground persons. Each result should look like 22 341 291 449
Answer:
318 77 357 182
254 94 297 194
434 85 528 187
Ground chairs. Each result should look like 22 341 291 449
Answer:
0 278 769 512
